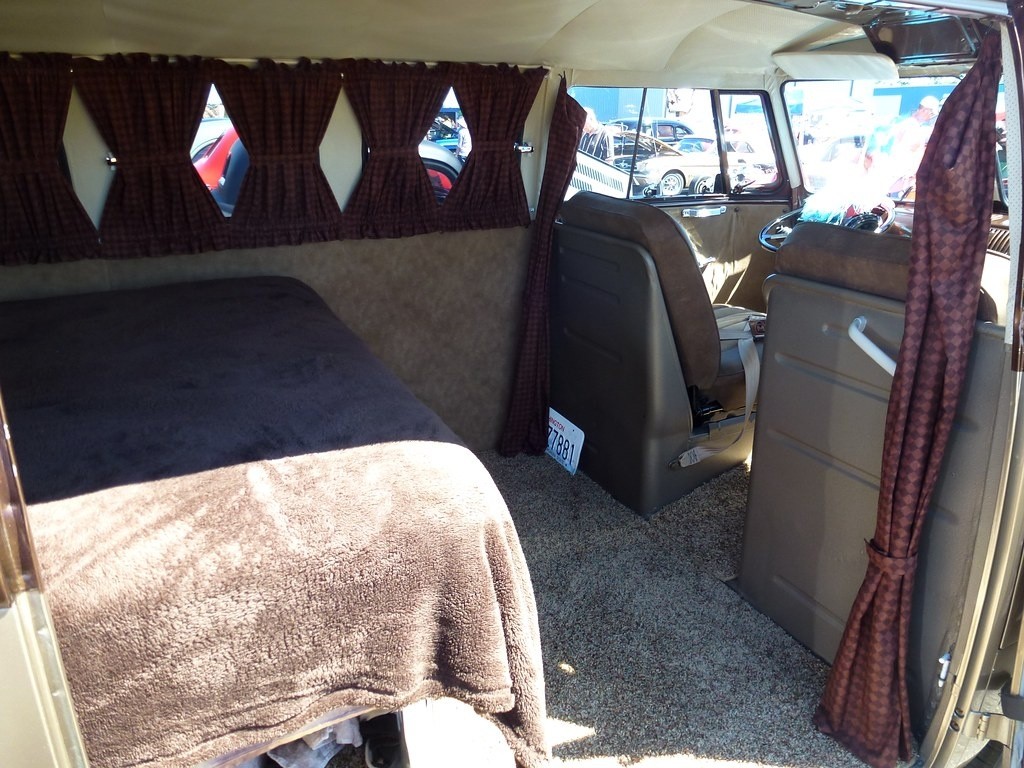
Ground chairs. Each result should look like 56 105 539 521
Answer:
739 221 1024 745
548 191 768 520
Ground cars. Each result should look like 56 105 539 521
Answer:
584 116 1008 203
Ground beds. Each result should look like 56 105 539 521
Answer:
0 275 552 768
